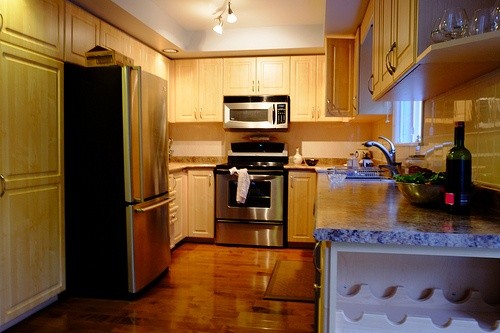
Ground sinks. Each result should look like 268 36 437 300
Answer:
344 176 381 182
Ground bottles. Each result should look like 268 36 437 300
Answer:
346 153 358 171
405 153 428 173
293 147 303 165
442 121 472 215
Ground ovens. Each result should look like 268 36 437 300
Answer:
214 169 287 248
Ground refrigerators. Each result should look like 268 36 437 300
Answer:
64 64 172 300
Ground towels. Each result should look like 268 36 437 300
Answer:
228 166 251 204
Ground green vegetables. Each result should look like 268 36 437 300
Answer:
393 172 445 184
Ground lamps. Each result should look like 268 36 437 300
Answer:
227 2 236 23
213 15 223 35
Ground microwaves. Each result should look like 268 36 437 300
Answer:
223 101 289 129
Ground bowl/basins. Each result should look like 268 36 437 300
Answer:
395 181 444 209
305 159 319 166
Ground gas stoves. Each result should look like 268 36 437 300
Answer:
215 142 289 169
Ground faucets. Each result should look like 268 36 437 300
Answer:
364 136 401 178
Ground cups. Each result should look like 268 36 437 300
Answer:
431 6 500 43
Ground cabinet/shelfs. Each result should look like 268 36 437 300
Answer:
0 0 172 326
169 171 186 247
290 28 394 123
167 59 224 124
223 56 290 96
368 0 500 101
188 168 216 241
287 171 317 243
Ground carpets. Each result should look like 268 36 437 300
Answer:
263 259 315 302
